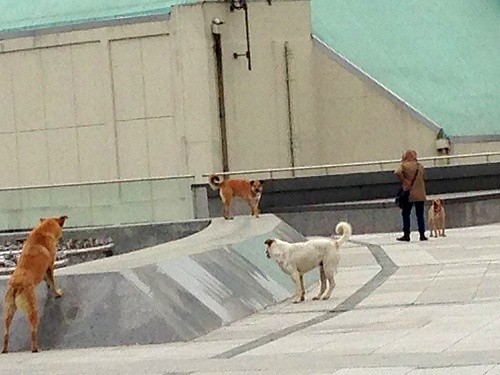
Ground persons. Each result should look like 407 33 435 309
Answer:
392 149 431 242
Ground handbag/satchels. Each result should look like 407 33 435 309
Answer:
395 187 410 208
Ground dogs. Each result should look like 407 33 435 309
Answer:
209 175 265 219
428 199 448 238
1 215 69 353
265 221 353 304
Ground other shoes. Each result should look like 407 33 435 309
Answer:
421 237 428 240
397 236 410 241
